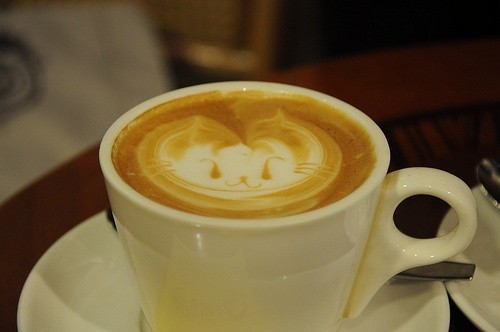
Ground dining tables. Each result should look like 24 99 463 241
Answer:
1 40 500 331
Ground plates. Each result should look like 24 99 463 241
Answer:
437 185 500 331
15 207 452 332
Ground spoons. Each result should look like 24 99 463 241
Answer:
477 155 499 211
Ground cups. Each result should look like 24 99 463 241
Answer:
100 81 477 332
